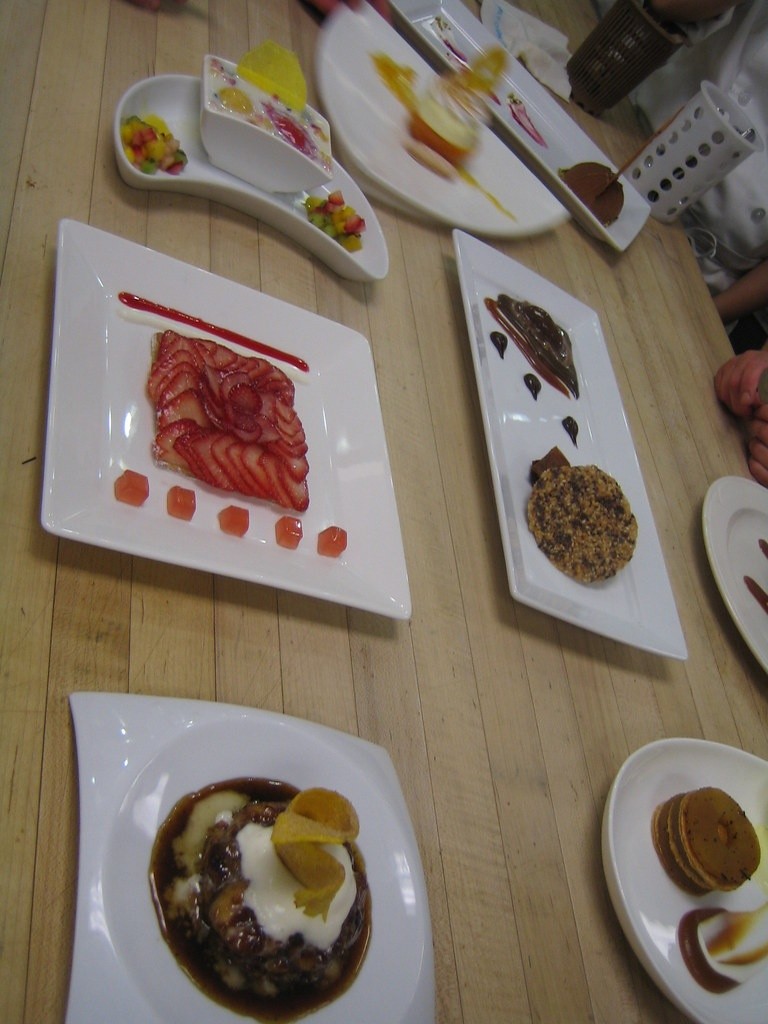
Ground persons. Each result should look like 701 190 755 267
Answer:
715 341 768 487
598 0 767 334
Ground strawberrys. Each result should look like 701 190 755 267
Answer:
147 329 311 513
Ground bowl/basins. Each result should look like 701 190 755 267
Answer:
199 54 334 194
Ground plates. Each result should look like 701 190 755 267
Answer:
452 228 688 661
702 475 768 675
112 75 389 283
64 692 437 1024
389 0 651 251
601 737 768 1024
314 1 572 240
40 217 412 621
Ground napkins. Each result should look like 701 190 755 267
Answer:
479 0 571 103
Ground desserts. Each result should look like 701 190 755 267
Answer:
555 104 685 228
411 92 475 166
527 445 637 585
651 786 761 896
679 900 768 993
148 776 372 1024
203 40 334 182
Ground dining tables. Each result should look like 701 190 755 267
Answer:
0 0 768 1024
